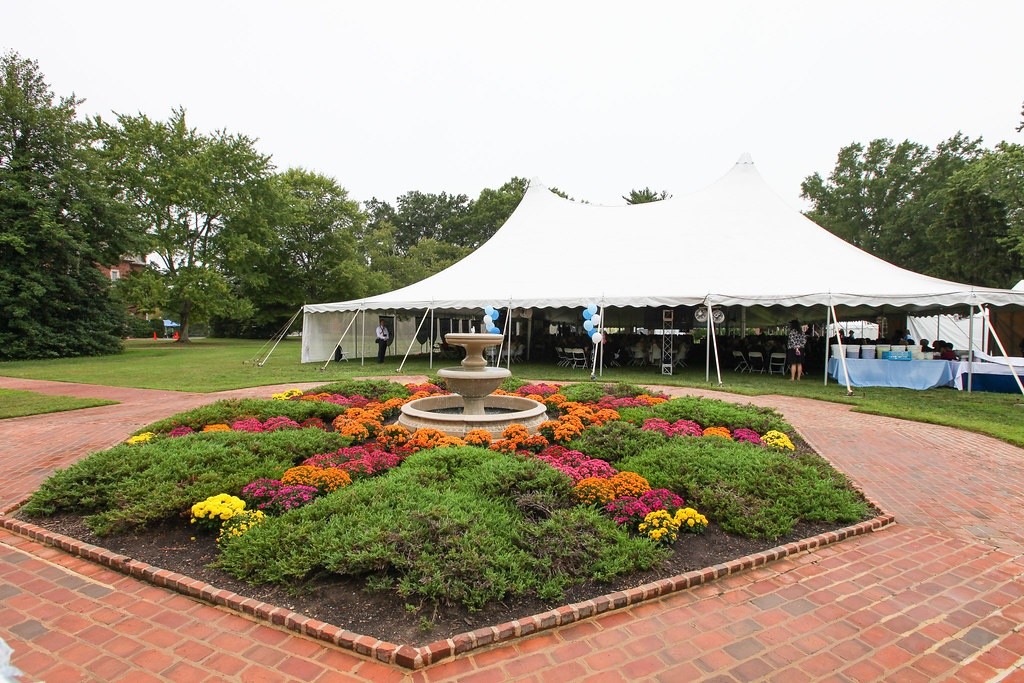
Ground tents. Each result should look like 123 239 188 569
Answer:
246 175 1024 399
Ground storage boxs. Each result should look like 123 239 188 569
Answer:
882 351 911 361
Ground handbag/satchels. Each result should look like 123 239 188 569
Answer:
375 339 379 343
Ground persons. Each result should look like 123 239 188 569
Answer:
498 328 956 369
376 319 389 364
786 320 807 383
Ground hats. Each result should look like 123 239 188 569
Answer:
839 329 844 334
849 330 854 334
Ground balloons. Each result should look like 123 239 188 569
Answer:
484 305 500 335
582 301 602 343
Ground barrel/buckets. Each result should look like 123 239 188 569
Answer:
861 345 876 359
830 344 835 357
907 345 922 360
834 345 847 359
877 345 891 358
891 345 907 351
847 345 860 359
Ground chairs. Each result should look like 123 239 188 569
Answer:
337 346 349 362
609 347 688 369
484 343 527 367
732 350 786 376
553 348 588 370
